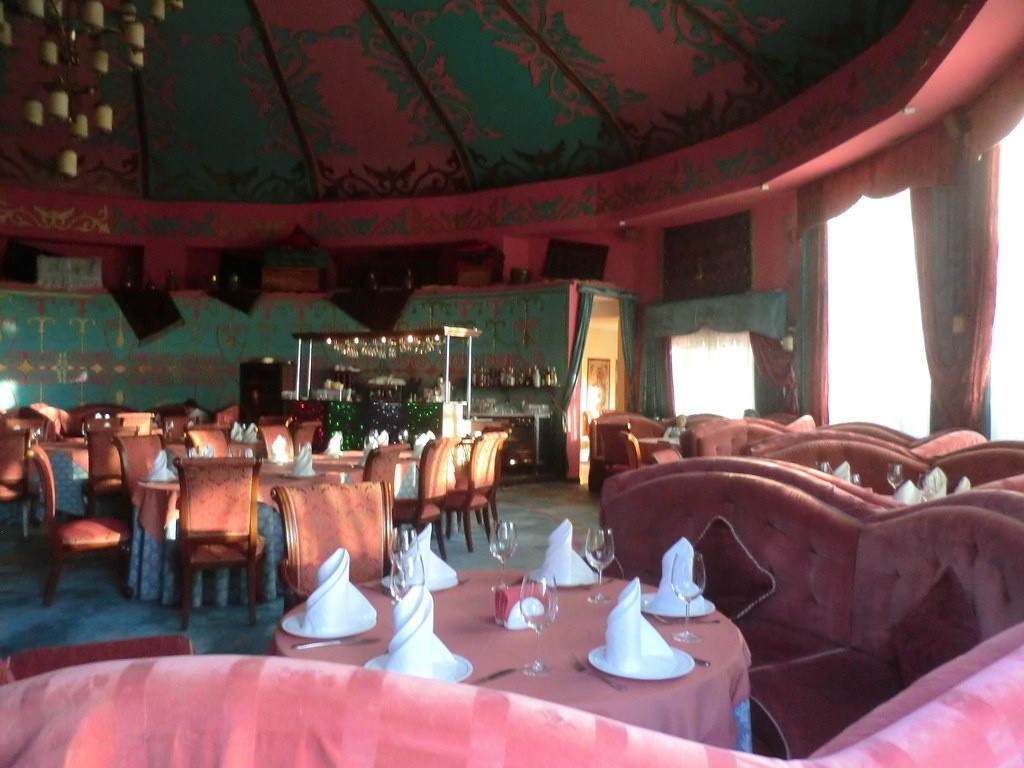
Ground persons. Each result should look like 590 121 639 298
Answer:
431 374 445 395
663 414 687 438
183 399 209 417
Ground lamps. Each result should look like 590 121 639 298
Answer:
0 0 183 179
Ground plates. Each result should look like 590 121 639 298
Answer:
636 590 716 619
527 567 600 588
361 650 474 683
587 645 695 681
279 471 320 480
281 612 377 641
380 573 459 593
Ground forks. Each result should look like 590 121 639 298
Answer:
651 610 720 627
567 650 627 694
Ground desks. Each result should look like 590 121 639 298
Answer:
268 570 753 754
35 436 91 520
128 465 350 608
639 437 681 457
284 450 419 550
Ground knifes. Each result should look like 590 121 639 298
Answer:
293 637 383 650
465 667 519 686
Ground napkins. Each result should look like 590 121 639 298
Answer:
271 434 288 463
401 521 456 585
300 546 379 632
923 466 947 500
363 441 379 467
386 585 453 674
604 577 673 665
835 461 850 482
148 448 174 481
293 442 315 475
376 429 389 446
412 430 436 458
895 480 923 505
231 422 244 442
245 422 258 442
655 536 705 609
954 475 971 493
543 520 594 583
325 430 343 456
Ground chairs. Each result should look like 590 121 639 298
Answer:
1 399 512 630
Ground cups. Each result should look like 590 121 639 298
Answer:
398 428 409 445
818 459 831 475
226 442 255 458
388 523 425 605
469 397 550 415
850 472 861 488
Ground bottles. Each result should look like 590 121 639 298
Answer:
472 364 557 389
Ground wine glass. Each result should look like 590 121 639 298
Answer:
30 425 42 447
518 570 560 677
359 434 376 468
165 419 174 444
488 518 518 594
81 421 88 449
917 472 939 505
886 462 905 498
584 526 616 604
670 552 707 644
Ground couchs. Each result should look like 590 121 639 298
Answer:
1 634 781 768
586 413 1024 768
